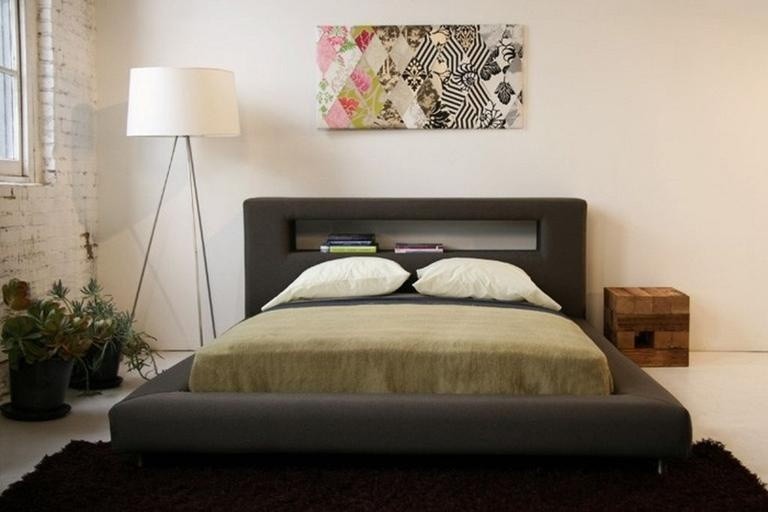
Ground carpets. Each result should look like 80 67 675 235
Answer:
0 436 766 512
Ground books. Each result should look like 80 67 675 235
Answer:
316 231 381 255
393 241 445 254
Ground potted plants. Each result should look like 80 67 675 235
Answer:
60 279 162 386
0 275 95 419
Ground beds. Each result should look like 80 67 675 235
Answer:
105 195 693 461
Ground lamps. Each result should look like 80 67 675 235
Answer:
125 65 245 362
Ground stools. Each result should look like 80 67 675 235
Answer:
602 283 689 370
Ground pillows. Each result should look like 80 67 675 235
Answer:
414 257 563 311
261 257 409 310
